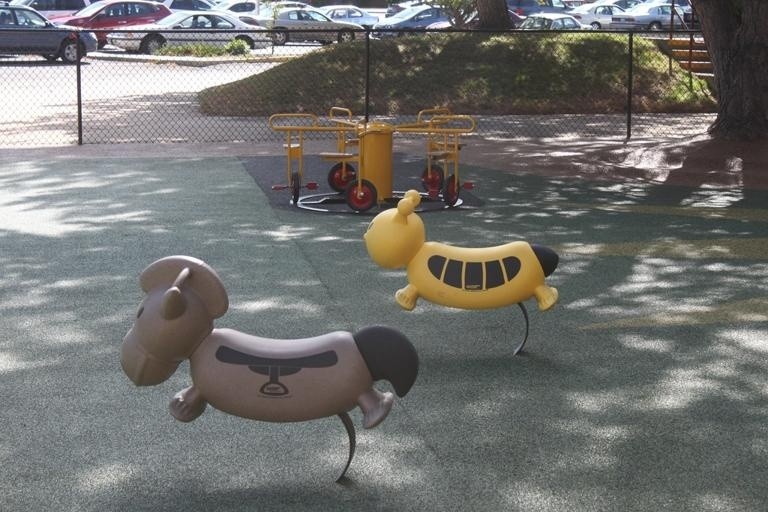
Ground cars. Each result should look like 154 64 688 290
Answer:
371 5 458 39
319 5 379 32
0 5 99 64
238 7 369 47
50 0 173 51
106 9 269 55
426 0 684 37
